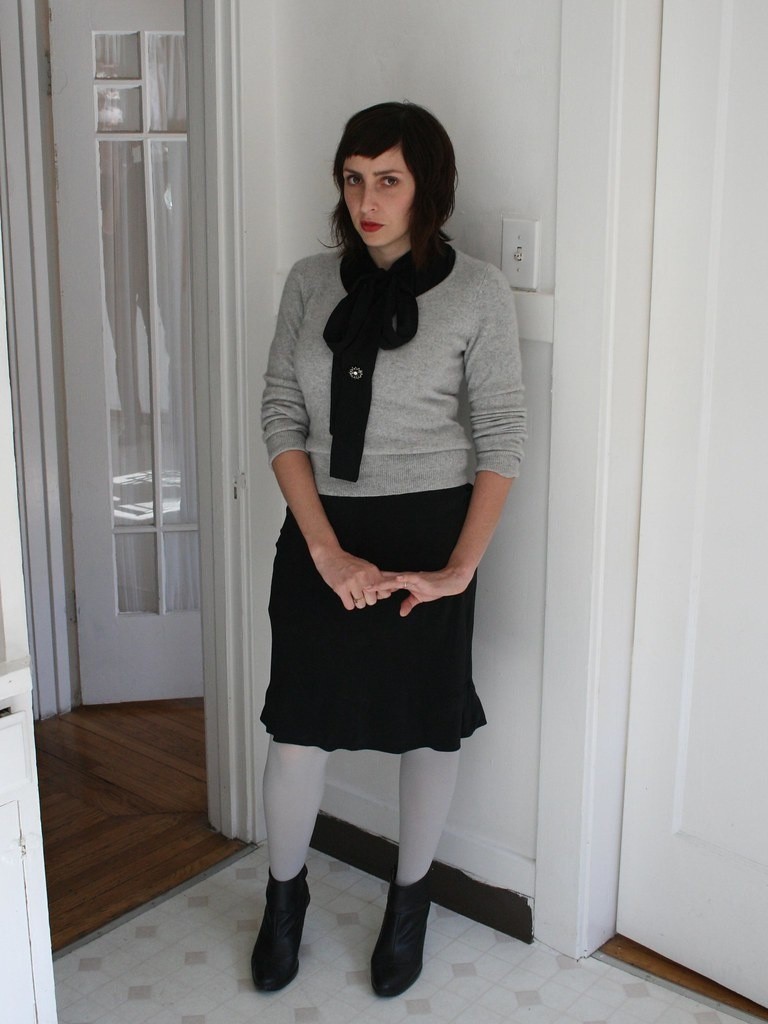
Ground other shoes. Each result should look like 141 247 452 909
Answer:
119 427 143 444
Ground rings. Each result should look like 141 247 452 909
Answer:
355 597 364 603
404 583 406 589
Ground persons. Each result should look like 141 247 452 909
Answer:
252 101 526 998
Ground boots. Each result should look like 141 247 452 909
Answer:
251 862 310 991
371 868 431 997
